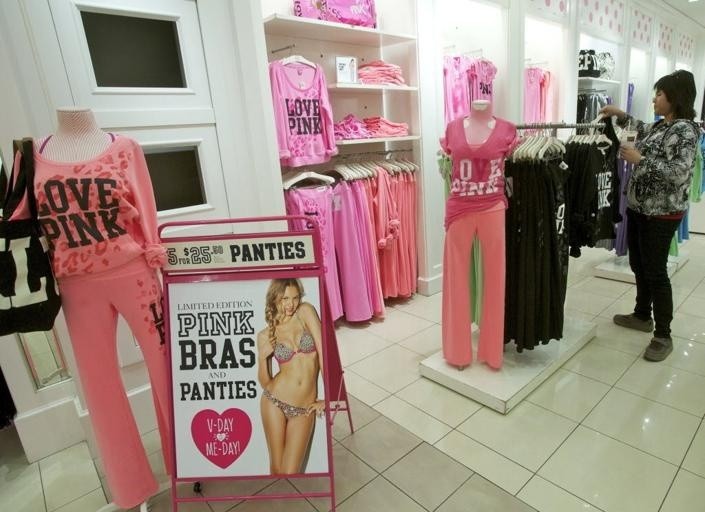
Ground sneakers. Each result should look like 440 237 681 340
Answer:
613 313 654 332
643 335 674 362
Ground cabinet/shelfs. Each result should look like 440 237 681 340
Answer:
264 13 420 145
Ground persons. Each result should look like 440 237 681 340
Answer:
438 100 519 369
599 68 700 362
257 278 326 477
1 107 191 512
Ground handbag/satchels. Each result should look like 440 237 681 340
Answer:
0 215 62 337
577 49 615 79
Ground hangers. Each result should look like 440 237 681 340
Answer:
279 44 316 70
512 119 612 162
527 61 550 81
578 88 608 99
443 44 489 63
283 148 420 190
